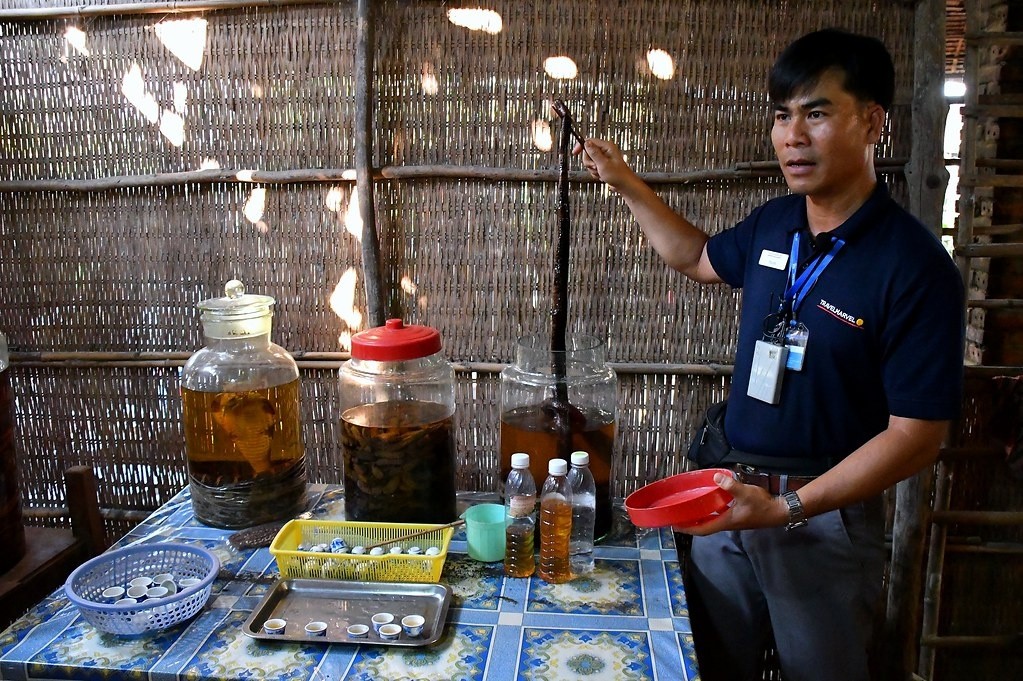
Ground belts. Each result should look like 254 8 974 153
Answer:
733 463 819 495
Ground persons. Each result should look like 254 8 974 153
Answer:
573 27 966 681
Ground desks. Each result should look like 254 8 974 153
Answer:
0 485 702 681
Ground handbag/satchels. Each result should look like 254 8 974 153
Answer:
685 399 838 469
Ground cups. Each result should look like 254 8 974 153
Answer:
264 619 287 634
379 624 402 641
297 538 440 578
347 624 369 638
305 622 327 637
402 615 425 637
459 503 509 561
371 613 394 635
102 573 201 628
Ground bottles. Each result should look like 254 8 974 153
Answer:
181 280 307 529
0 331 27 575
338 319 458 525
566 451 596 575
499 332 618 544
538 459 572 584
505 453 537 578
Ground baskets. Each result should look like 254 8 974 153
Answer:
64 542 217 634
269 520 453 583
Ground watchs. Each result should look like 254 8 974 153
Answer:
784 491 808 533
